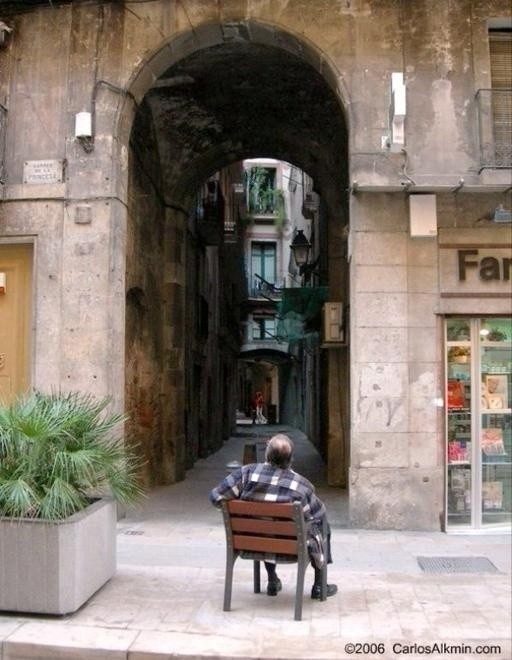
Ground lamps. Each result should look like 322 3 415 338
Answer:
289 228 319 283
75 111 91 143
478 318 491 336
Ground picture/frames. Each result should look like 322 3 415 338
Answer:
482 373 512 410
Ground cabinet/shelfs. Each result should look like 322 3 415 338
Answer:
443 314 512 535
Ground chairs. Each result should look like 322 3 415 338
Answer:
219 495 328 620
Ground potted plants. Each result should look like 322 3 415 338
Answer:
0 388 152 615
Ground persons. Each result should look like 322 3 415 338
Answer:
256 391 265 424
209 434 337 599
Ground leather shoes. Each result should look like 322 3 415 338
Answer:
310 584 338 600
267 578 282 596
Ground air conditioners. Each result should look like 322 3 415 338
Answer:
322 300 345 344
208 180 223 203
